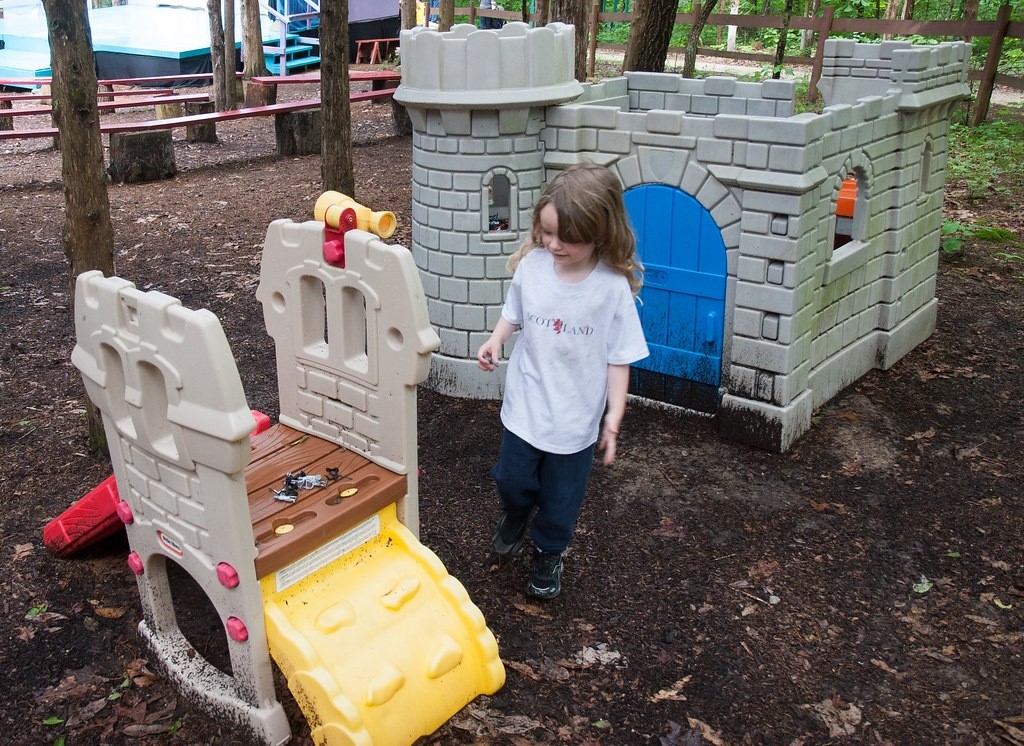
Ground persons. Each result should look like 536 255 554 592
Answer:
477 162 649 599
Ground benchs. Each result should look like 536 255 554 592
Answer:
356 38 401 65
0 71 402 182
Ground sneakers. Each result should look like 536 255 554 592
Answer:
527 548 564 599
491 513 530 557
